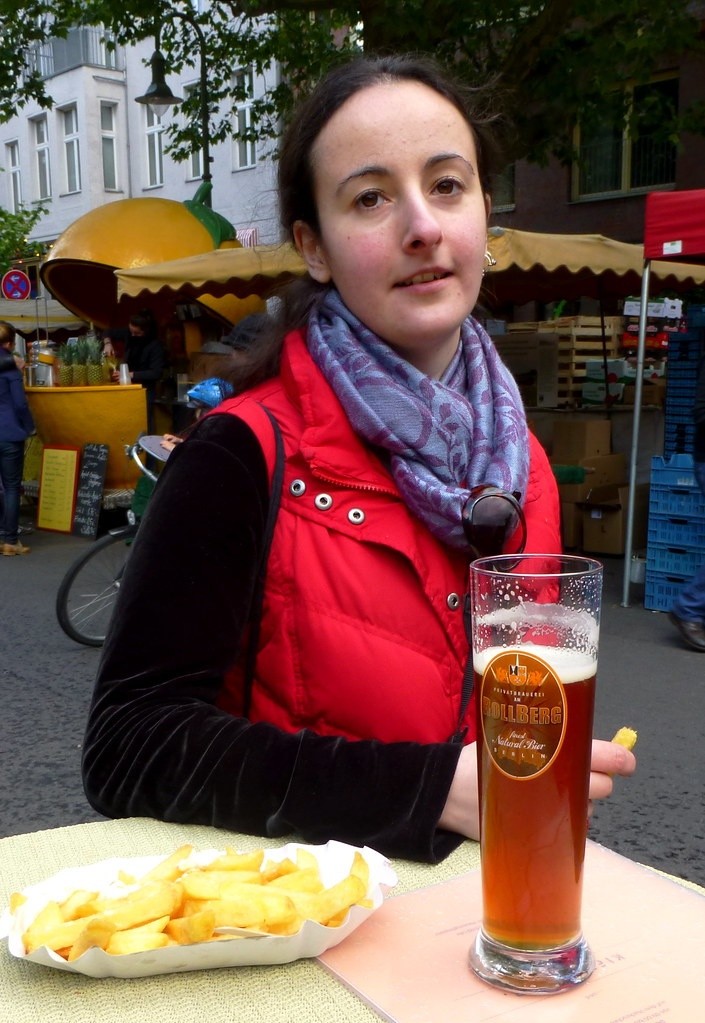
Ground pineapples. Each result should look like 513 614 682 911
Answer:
59 340 103 385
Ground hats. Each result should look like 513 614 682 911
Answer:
222 314 275 353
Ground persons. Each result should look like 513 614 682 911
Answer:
652 326 705 650
0 320 37 556
186 312 282 420
81 54 638 867
101 314 166 471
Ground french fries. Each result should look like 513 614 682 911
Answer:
22 846 372 960
603 726 637 778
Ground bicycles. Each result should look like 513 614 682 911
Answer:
57 432 160 646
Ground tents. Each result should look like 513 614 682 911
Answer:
620 188 705 611
0 296 85 334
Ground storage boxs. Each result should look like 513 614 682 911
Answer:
523 295 705 628
188 351 233 382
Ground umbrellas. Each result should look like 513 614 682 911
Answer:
112 228 705 303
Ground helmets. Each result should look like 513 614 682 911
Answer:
188 377 233 411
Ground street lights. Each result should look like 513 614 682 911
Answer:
134 12 213 207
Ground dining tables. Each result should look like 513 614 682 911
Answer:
0 794 705 1023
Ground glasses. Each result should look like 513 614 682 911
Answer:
461 484 532 646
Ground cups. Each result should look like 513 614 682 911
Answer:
24 367 36 387
466 552 605 997
45 365 56 386
119 364 131 385
177 374 189 404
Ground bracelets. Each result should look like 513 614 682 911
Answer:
104 342 112 344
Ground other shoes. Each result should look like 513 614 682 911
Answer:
1 537 31 556
